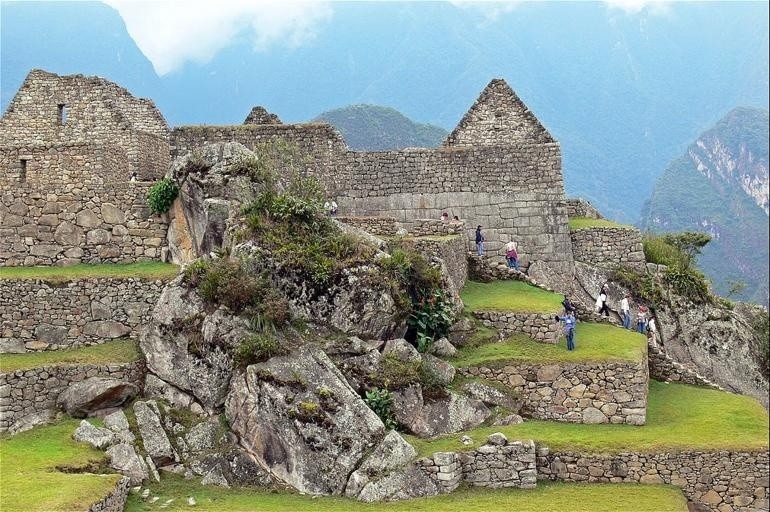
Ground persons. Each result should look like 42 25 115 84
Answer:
648 315 658 349
635 303 650 331
595 278 613 309
322 196 339 217
555 294 576 350
476 225 486 257
439 212 449 221
621 294 632 329
129 171 166 182
451 215 460 223
506 239 520 270
598 283 610 318
636 309 646 335
